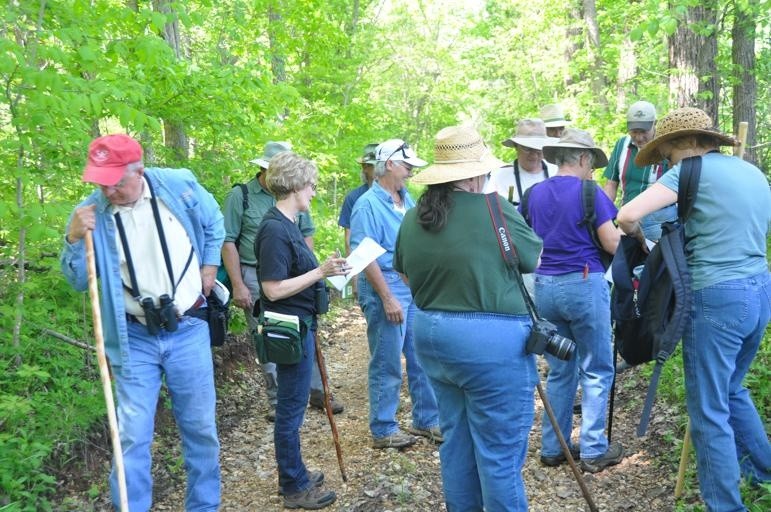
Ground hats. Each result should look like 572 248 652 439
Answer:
82 135 142 186
249 141 291 170
355 100 741 185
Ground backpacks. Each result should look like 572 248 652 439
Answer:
611 156 701 365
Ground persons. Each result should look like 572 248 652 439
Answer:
351 138 444 449
220 141 343 420
336 143 380 300
517 126 627 470
601 101 679 373
483 118 557 300
613 105 770 509
388 118 548 512
392 125 543 512
60 136 228 511
256 150 352 507
539 103 572 165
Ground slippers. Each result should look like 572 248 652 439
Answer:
611 156 701 365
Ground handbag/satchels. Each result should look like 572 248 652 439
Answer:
217 264 233 299
253 314 308 365
202 289 231 346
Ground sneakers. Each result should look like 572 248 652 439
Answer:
278 471 324 495
310 396 343 413
283 487 336 506
581 442 621 472
269 405 277 420
372 431 416 448
540 443 580 465
408 423 442 442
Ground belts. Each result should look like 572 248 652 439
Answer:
126 313 138 322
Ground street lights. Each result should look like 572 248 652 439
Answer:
202 289 231 346
253 314 308 365
217 264 233 299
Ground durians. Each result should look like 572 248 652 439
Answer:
142 294 178 335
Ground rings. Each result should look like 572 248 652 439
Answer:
336 248 348 281
399 323 404 339
584 261 589 280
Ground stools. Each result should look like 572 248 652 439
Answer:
126 313 138 322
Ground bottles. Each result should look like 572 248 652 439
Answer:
249 141 291 170
82 135 142 186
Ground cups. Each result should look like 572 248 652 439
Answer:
308 182 316 191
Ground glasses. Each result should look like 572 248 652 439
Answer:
308 182 316 191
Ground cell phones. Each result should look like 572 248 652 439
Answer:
526 319 576 363
